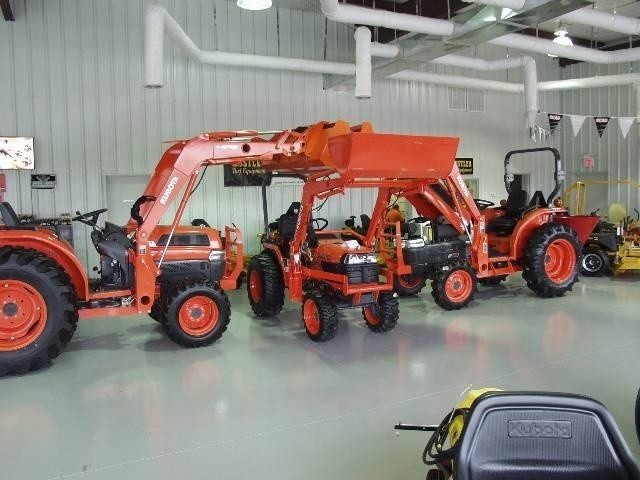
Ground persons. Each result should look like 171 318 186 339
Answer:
384 205 405 226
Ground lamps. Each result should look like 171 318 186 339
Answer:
235 0 273 12
548 24 575 60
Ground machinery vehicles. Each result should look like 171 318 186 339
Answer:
1 118 350 377
246 131 462 342
350 121 598 309
569 180 640 278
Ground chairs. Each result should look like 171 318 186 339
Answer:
0 201 61 237
278 212 320 263
486 188 531 238
